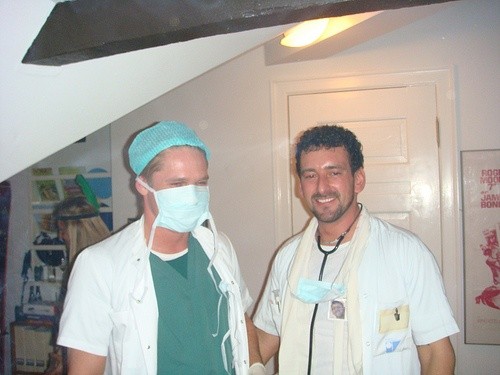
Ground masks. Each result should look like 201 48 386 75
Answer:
135 177 209 232
294 279 342 304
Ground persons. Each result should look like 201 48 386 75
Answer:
330 301 345 319
56 121 267 375
251 124 460 375
